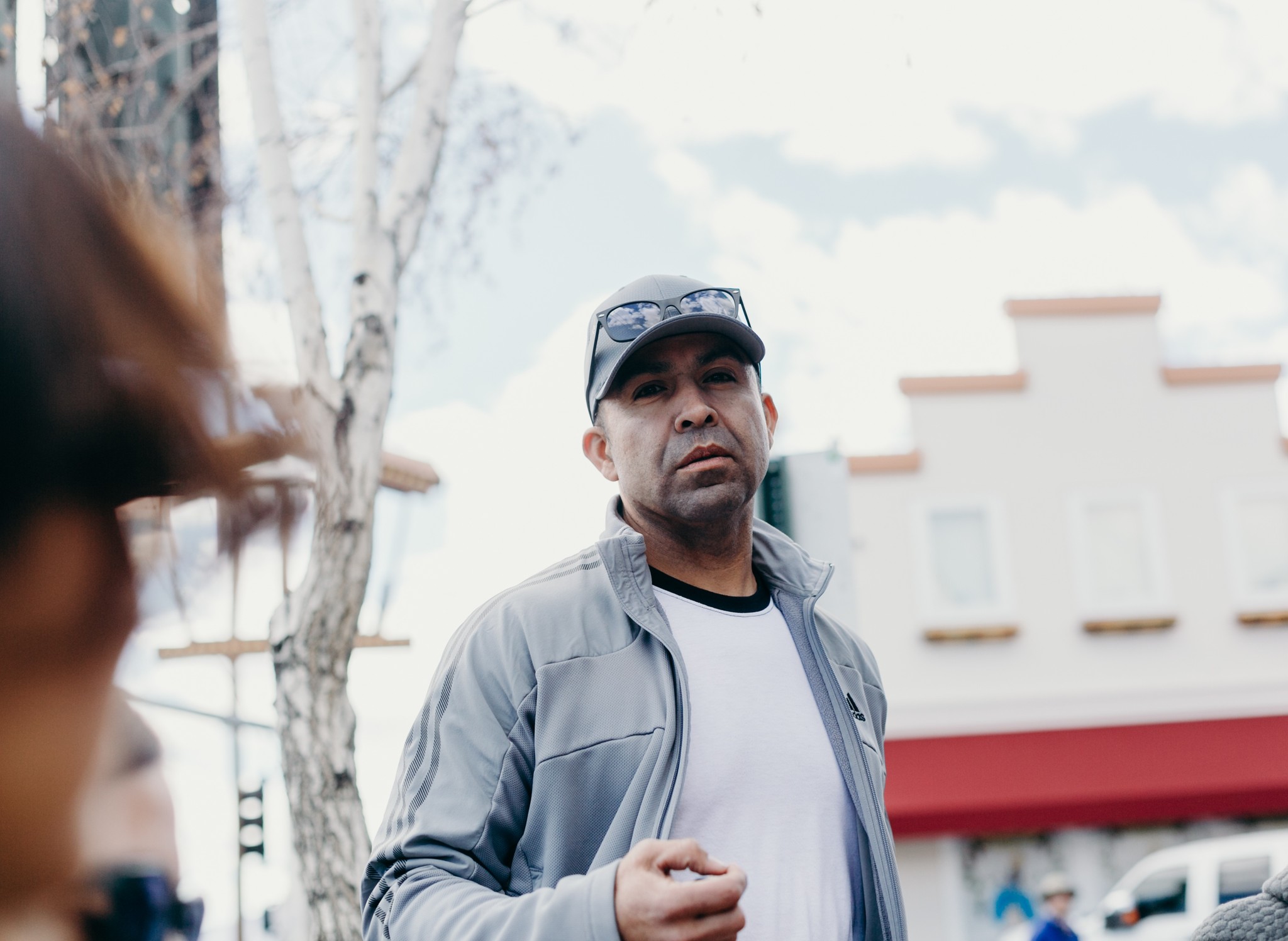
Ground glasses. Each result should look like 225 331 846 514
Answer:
583 288 752 426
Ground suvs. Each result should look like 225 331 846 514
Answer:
1011 827 1286 941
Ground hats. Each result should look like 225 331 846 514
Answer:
587 273 766 423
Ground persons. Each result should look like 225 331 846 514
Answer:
3 99 288 941
1028 875 1081 941
360 272 911 941
